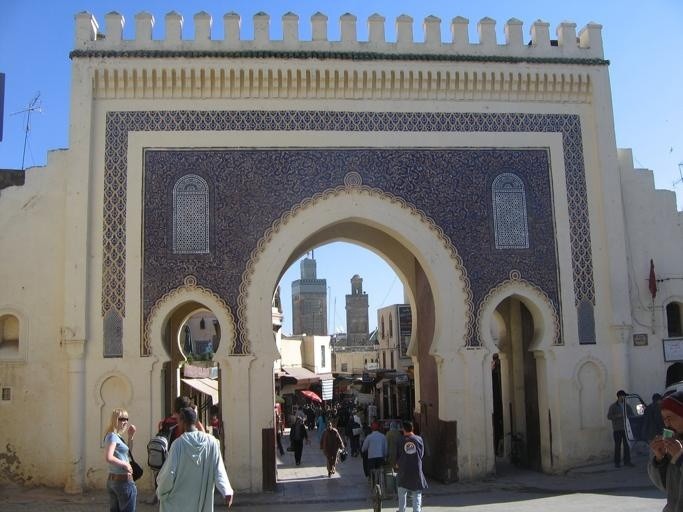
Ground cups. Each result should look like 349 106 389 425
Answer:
661 429 674 441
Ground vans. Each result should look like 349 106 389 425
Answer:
620 378 682 441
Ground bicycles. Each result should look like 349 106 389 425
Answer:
367 466 383 512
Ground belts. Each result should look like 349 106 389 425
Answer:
108 473 133 481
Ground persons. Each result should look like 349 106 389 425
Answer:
158 396 206 451
607 391 683 511
209 415 220 432
155 407 235 512
103 408 137 512
274 401 429 512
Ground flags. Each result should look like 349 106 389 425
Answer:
649 260 657 299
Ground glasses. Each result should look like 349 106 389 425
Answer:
118 416 128 421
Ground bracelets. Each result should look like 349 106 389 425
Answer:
130 437 134 441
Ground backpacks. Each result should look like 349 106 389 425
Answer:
145 428 173 471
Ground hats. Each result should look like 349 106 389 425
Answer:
369 421 379 431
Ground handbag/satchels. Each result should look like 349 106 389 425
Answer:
339 448 349 463
129 460 143 482
